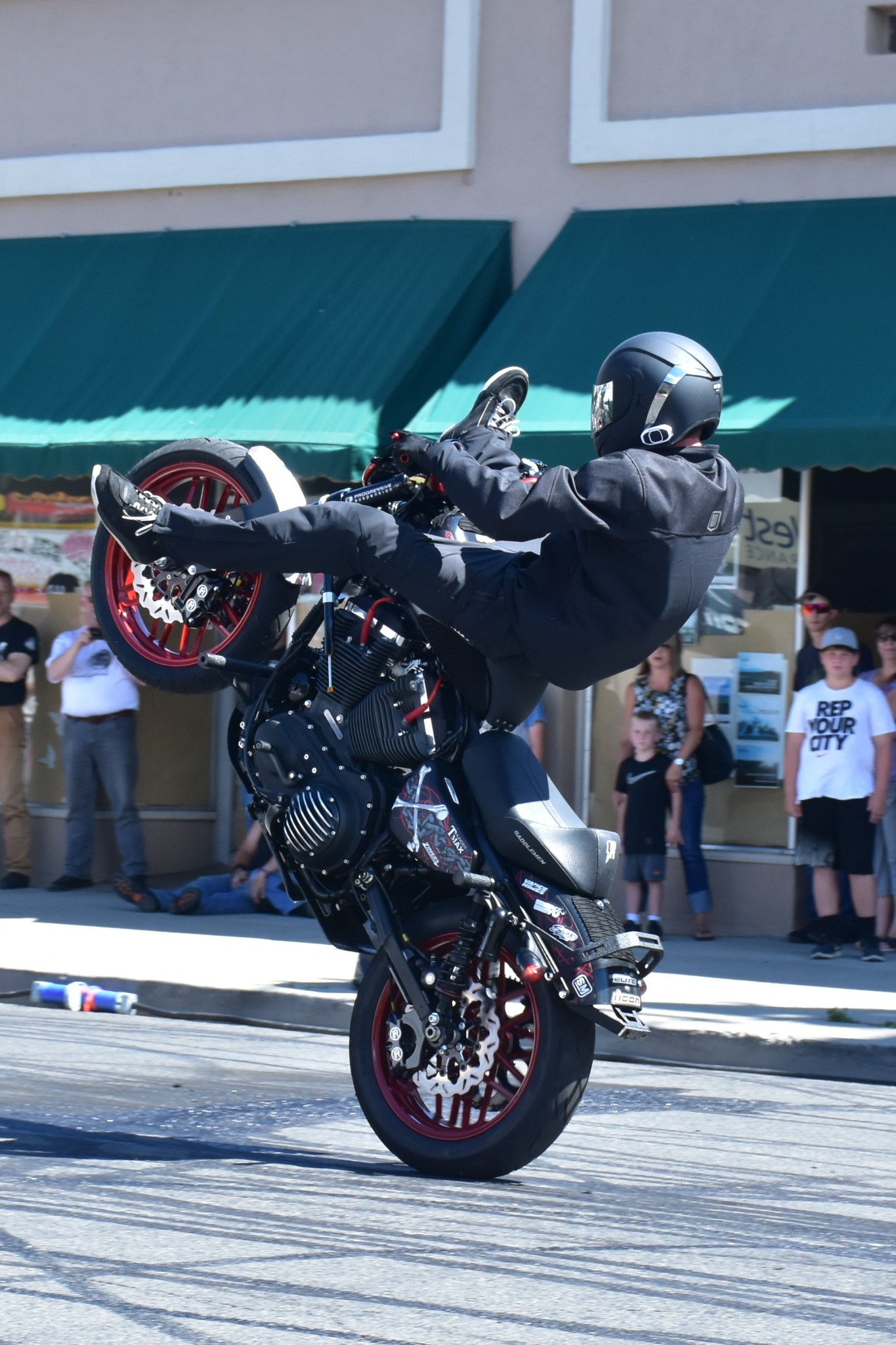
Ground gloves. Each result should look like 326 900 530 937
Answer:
394 435 428 475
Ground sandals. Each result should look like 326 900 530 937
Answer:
856 937 896 952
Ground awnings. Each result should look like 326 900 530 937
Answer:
1 220 512 490
402 195 896 470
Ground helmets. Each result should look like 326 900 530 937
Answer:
591 331 724 457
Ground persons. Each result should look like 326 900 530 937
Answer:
0 569 40 889
855 619 896 952
783 628 896 962
92 332 743 692
510 696 548 762
786 588 875 945
44 582 150 893
613 710 685 940
113 821 310 917
612 633 713 939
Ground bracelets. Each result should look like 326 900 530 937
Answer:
232 864 249 872
258 867 268 875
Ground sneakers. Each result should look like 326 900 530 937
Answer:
437 365 530 444
91 464 168 565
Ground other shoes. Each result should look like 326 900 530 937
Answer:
622 919 642 933
647 919 663 939
112 872 159 912
810 941 844 959
862 946 884 960
171 887 201 916
50 876 92 892
0 873 30 889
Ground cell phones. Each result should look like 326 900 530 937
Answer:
87 627 104 639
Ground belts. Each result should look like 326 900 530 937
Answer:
69 709 133 725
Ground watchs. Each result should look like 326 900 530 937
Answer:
673 758 687 770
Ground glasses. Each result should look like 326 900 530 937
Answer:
878 631 896 643
661 641 672 650
799 602 828 615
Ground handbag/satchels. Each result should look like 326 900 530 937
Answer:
680 672 732 783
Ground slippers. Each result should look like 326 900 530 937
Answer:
693 927 715 940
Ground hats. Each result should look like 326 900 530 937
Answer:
795 585 837 607
819 627 859 654
